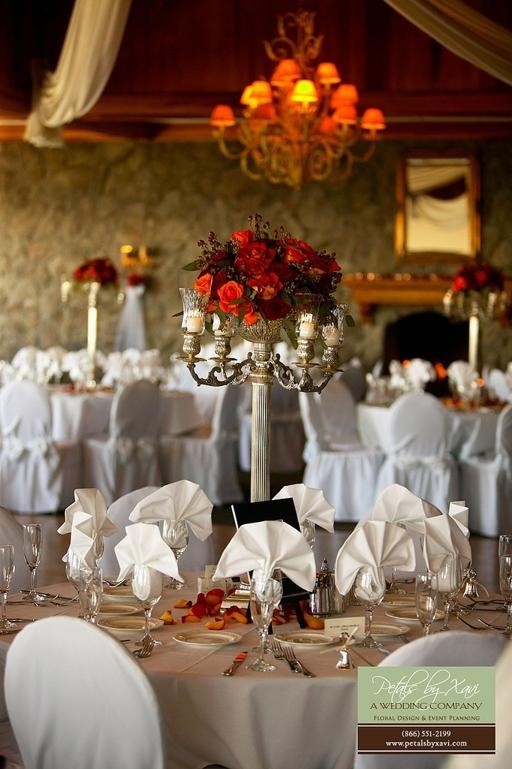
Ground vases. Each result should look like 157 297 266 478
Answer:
178 288 350 606
444 290 506 370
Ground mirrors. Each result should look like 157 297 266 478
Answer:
395 147 483 258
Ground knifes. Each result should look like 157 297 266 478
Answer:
223 650 247 679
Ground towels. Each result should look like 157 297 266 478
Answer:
15 343 161 377
385 358 405 386
406 360 429 385
271 483 337 541
490 368 510 403
57 486 118 560
113 522 185 601
449 500 470 538
447 359 477 389
63 509 99 584
372 483 426 537
129 479 213 548
335 519 416 603
424 514 470 592
212 519 317 601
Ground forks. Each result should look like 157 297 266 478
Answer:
124 636 157 660
283 647 316 678
271 642 301 673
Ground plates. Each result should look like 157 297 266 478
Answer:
273 631 340 646
174 630 242 646
370 622 413 636
102 587 136 601
386 607 445 623
98 604 142 614
98 616 164 634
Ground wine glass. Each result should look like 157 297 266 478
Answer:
132 566 164 647
80 568 102 622
432 561 463 631
246 580 282 671
65 555 98 618
0 544 18 628
415 574 438 635
162 519 189 589
500 535 512 607
22 523 44 601
352 568 385 649
500 554 512 634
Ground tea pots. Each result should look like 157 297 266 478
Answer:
309 575 331 617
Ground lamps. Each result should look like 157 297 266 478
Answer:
211 9 385 190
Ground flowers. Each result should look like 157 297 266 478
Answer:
72 256 119 288
178 213 356 351
120 241 149 289
444 258 506 295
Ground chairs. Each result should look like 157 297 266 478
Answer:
299 392 376 523
82 379 159 508
166 382 243 507
95 486 214 578
461 403 511 538
375 390 458 513
3 615 163 769
239 369 304 475
0 380 64 515
321 381 357 451
357 630 509 769
